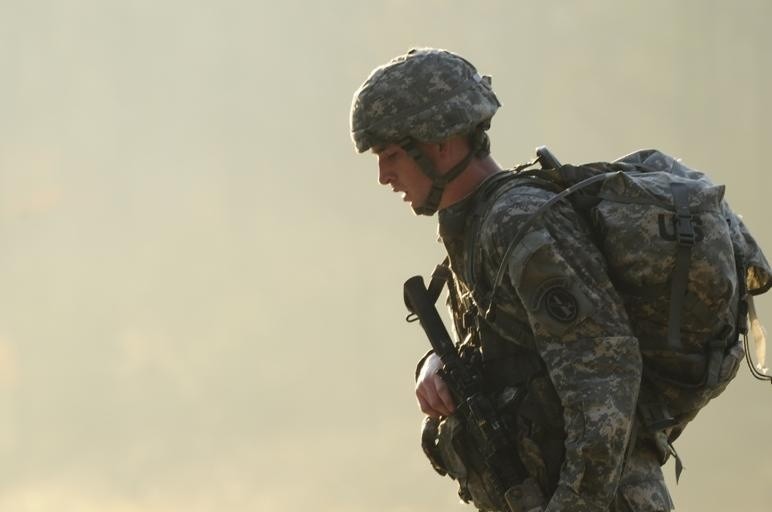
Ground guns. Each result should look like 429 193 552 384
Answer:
403 274 547 511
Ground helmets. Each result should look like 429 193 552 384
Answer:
351 48 500 154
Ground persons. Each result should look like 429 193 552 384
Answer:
350 49 673 511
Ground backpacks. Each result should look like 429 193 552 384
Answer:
520 145 770 466
